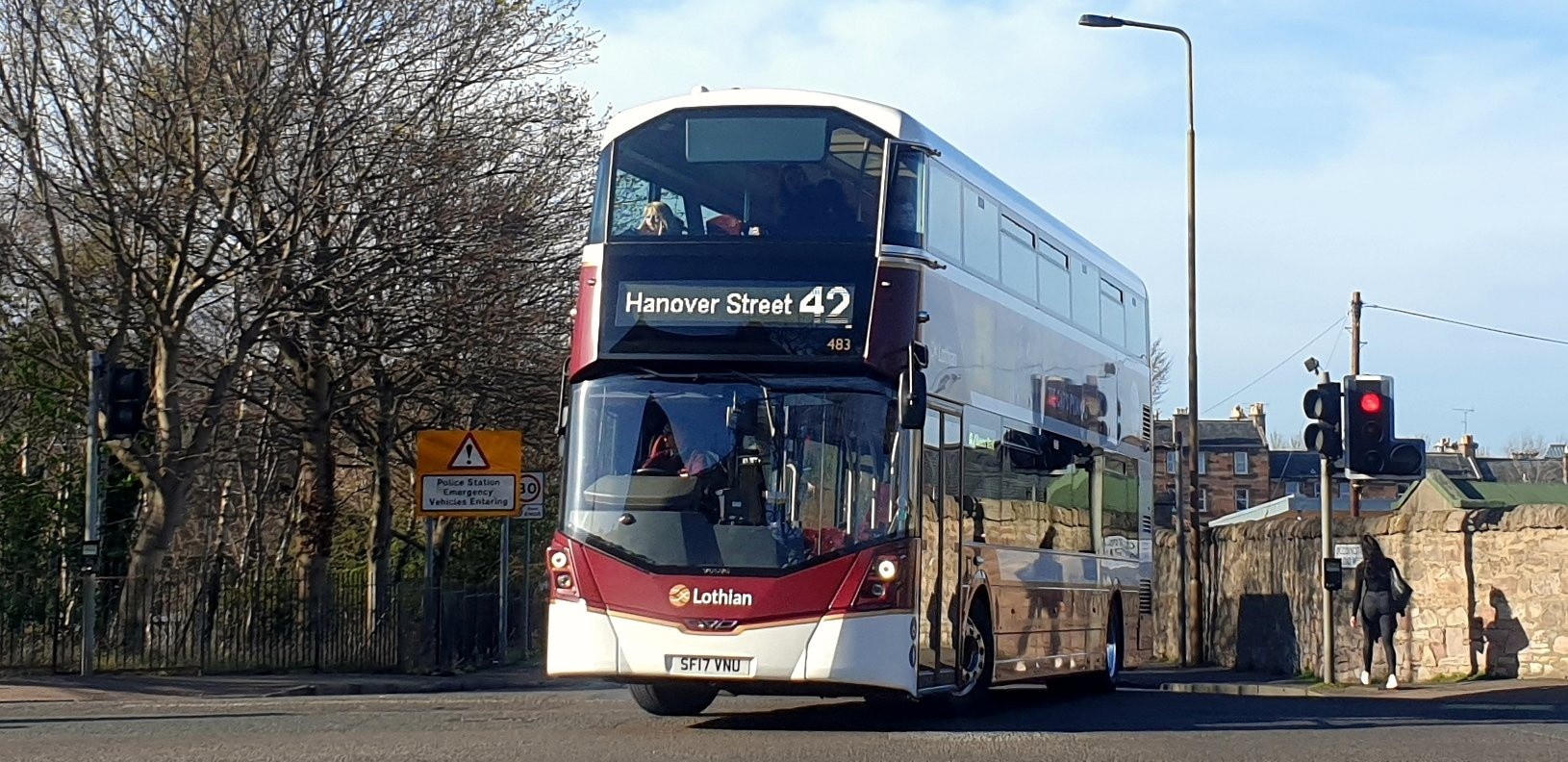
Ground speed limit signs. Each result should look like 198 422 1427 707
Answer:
520 474 540 502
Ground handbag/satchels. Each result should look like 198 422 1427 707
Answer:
1388 561 1407 601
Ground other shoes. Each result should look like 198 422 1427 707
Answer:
1361 670 1371 686
1385 674 1399 689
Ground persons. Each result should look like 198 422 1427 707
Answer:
1350 534 1406 689
770 165 845 229
1015 552 1066 672
634 202 684 236
642 418 703 477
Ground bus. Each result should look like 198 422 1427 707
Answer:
544 85 1155 715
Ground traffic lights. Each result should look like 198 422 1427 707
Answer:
1344 375 1426 482
1303 382 1343 461
104 360 147 440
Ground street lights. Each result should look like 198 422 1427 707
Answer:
1080 11 1205 670
